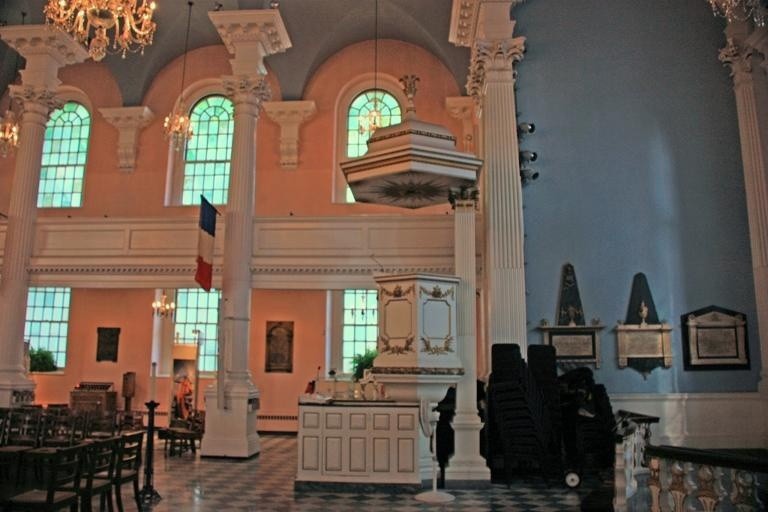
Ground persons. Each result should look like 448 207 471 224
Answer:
172 373 194 420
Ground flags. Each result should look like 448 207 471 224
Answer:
193 194 217 294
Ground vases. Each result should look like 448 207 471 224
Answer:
362 379 380 403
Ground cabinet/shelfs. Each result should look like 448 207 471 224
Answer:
170 343 199 424
70 390 117 412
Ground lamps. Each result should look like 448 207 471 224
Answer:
150 288 176 322
164 1 194 152
41 0 158 61
447 186 479 212
357 2 386 134
1 11 26 155
709 0 768 31
518 123 540 182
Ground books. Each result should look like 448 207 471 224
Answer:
300 394 334 406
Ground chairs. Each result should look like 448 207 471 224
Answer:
431 342 617 487
0 402 205 512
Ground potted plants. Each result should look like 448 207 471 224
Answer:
351 348 378 402
